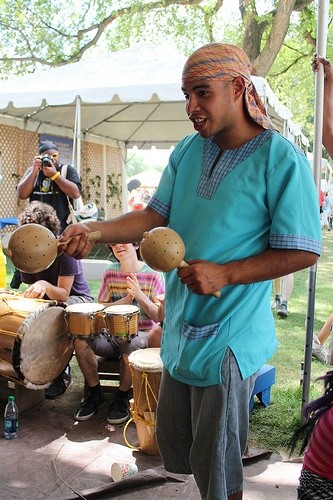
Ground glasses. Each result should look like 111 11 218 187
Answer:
49 151 59 160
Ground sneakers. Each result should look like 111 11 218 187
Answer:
45 372 74 399
73 382 106 420
107 389 131 424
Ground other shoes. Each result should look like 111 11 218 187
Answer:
271 296 288 319
311 342 333 366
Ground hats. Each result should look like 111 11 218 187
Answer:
39 142 58 154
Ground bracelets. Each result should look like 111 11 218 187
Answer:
50 171 60 181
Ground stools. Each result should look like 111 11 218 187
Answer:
249 364 277 413
81 354 121 394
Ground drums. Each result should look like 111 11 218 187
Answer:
102 304 140 343
64 302 106 340
123 347 164 456
0 288 75 390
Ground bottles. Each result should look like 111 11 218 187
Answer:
2 396 19 439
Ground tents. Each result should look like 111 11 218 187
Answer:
0 40 311 225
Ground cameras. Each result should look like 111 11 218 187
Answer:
39 154 53 166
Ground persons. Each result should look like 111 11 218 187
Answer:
16 140 83 236
55 42 333 500
18 200 95 399
